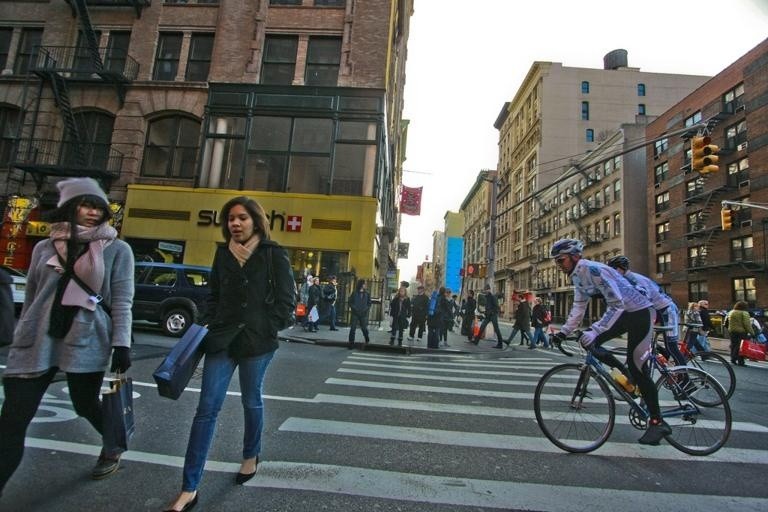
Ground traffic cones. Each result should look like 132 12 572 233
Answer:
474 315 480 336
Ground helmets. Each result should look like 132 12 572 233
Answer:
550 238 585 260
607 255 630 272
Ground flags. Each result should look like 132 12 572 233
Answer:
398 184 423 217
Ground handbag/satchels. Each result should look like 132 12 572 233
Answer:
103 365 137 454
152 321 211 399
262 248 297 331
737 337 767 363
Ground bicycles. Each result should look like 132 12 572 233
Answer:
535 323 731 458
599 319 738 406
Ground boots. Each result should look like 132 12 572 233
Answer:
389 336 395 344
397 338 404 346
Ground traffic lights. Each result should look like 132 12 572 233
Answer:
700 143 719 176
690 135 712 171
721 209 734 232
466 264 480 276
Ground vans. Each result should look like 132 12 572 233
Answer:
119 262 218 336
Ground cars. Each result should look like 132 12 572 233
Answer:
2 266 27 307
458 309 485 320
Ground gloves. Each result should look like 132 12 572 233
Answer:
575 326 600 349
109 345 132 375
552 332 566 348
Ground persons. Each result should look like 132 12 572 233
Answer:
546 236 675 447
599 256 697 399
691 300 716 360
725 301 755 362
746 312 761 335
163 192 297 512
299 275 553 351
0 175 135 495
681 299 705 355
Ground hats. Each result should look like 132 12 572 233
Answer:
55 175 111 209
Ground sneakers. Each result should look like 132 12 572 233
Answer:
637 420 673 447
416 337 422 341
674 377 690 394
407 337 414 340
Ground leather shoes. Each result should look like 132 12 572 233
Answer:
235 453 259 486
91 445 122 481
162 490 198 512
